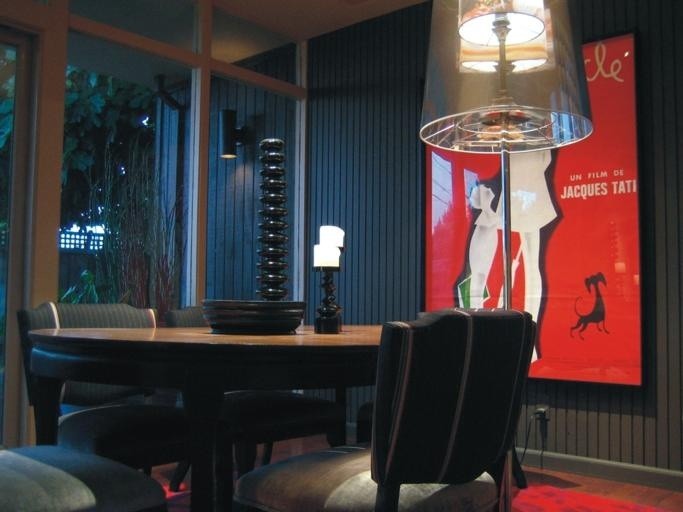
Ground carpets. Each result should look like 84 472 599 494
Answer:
511 483 667 512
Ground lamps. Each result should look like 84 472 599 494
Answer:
311 223 348 335
418 0 592 309
217 108 254 159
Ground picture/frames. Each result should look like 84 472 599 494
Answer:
420 28 650 392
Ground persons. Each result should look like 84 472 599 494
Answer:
495 147 559 363
467 184 499 309
